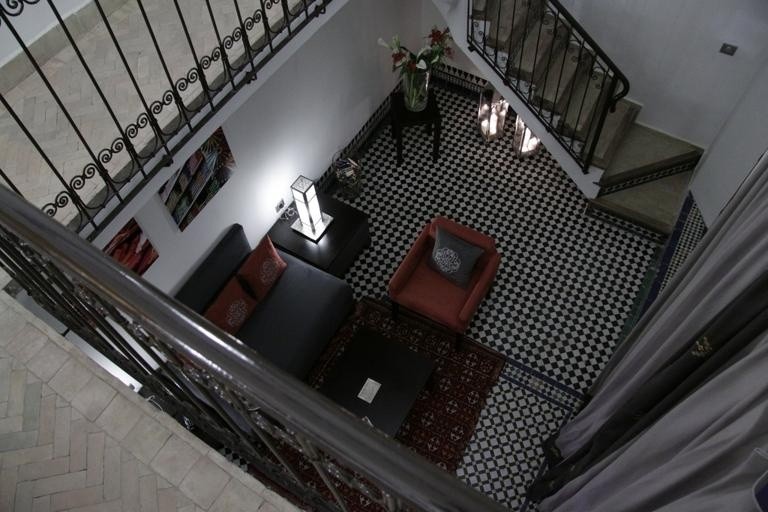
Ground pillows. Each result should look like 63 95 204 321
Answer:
202 233 288 336
425 224 485 291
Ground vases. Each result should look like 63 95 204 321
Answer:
402 72 429 113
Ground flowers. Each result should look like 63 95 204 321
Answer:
377 26 455 109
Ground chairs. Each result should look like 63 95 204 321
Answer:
388 215 502 353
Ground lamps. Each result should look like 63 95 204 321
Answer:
290 175 334 243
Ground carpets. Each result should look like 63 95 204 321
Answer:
247 295 507 511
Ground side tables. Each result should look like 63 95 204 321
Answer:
267 189 371 279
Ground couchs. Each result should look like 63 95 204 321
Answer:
173 224 353 381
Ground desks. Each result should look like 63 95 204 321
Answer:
389 88 442 164
